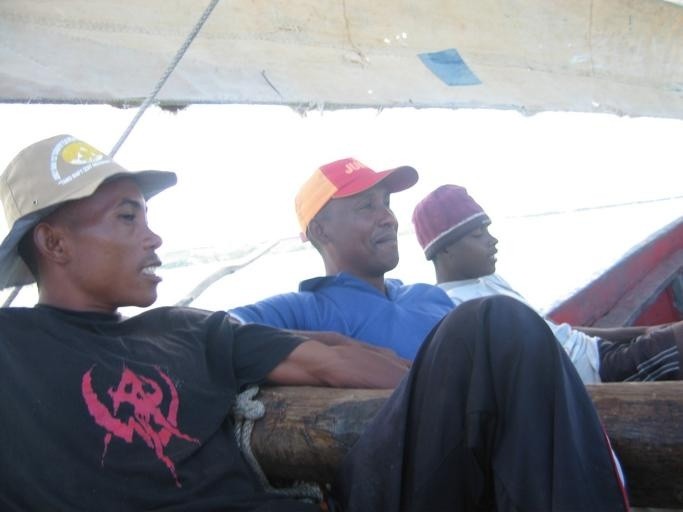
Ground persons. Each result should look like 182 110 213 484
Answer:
225 158 454 388
411 184 680 384
0 133 630 511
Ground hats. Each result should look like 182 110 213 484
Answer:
413 185 490 259
0 134 176 288
294 158 418 242
